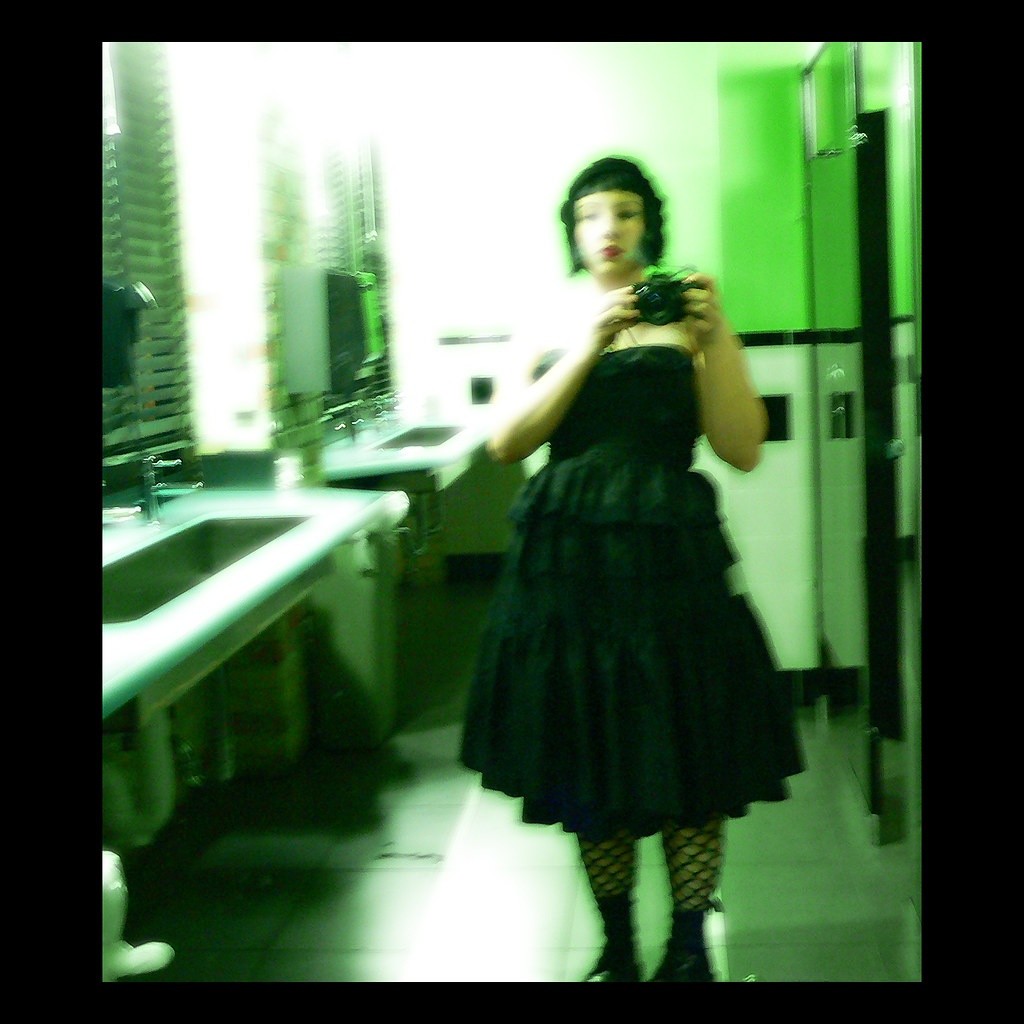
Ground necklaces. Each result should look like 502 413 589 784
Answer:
626 327 639 346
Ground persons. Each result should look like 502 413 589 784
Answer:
463 156 806 980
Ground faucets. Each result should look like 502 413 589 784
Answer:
135 450 207 523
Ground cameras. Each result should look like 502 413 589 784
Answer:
632 274 706 326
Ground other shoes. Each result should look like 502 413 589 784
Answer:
586 941 639 982
646 940 713 983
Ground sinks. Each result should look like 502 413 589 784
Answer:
372 424 468 451
102 510 313 629
